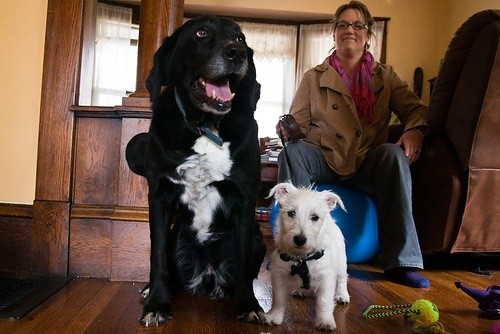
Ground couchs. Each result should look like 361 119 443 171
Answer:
389 9 500 264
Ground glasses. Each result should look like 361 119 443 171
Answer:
335 21 369 31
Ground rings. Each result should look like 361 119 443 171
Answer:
414 149 419 154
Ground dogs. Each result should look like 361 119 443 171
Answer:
139 16 267 329
264 179 351 331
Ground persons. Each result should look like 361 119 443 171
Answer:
276 1 432 288
